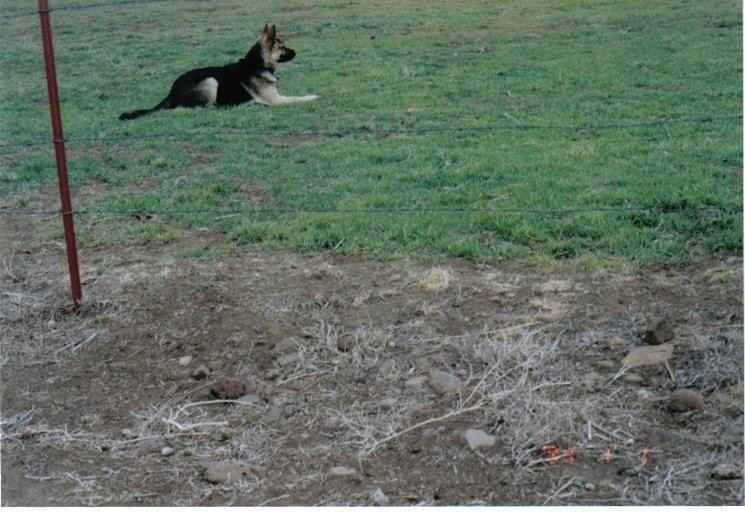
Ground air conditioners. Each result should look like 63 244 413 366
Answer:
118 22 322 121
